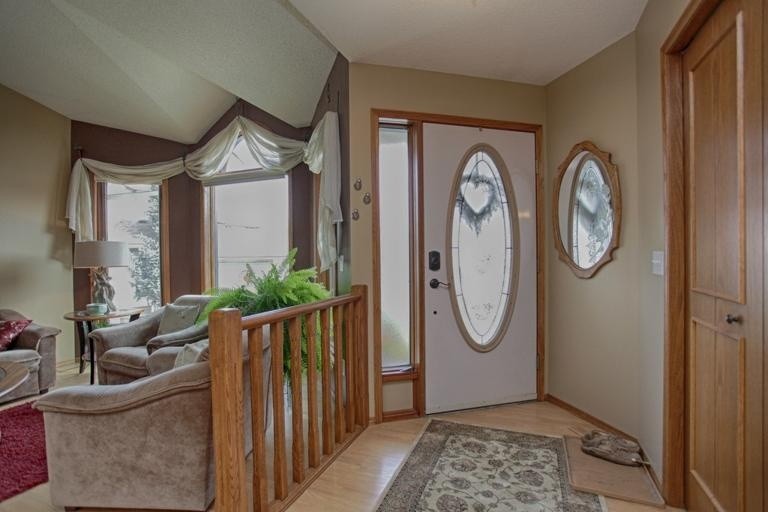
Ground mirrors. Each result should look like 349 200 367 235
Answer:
549 140 623 283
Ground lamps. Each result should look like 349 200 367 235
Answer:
73 236 130 314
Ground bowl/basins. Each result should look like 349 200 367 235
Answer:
86 303 108 316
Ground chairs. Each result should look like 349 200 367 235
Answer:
85 293 240 386
32 321 275 510
1 307 63 404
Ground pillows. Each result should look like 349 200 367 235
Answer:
155 302 201 337
1 317 33 354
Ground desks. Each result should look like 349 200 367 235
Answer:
64 306 148 387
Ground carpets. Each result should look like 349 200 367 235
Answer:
367 414 608 510
0 398 48 505
